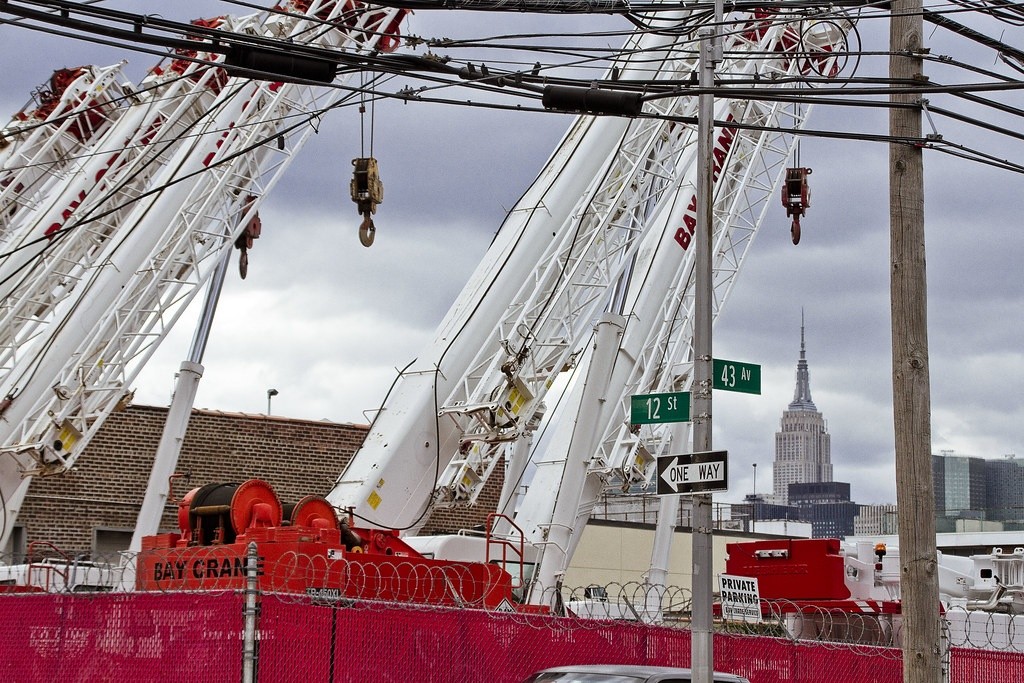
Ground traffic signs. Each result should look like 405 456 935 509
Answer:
655 449 729 498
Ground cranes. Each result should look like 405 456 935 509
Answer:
0 3 1022 683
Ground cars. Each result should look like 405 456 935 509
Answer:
520 663 749 682
